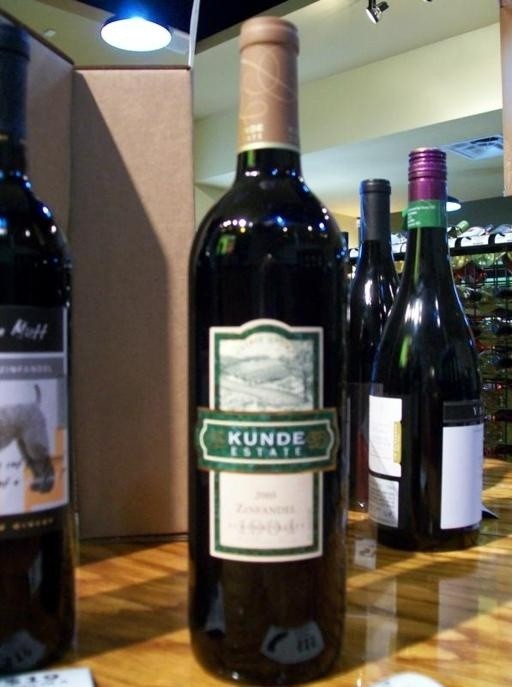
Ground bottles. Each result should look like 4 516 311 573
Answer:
0 23 77 678
346 178 402 512
188 16 348 687
368 147 482 552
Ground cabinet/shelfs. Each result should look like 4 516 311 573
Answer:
351 241 512 458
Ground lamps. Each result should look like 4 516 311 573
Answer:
101 0 173 52
446 196 461 212
364 0 388 23
